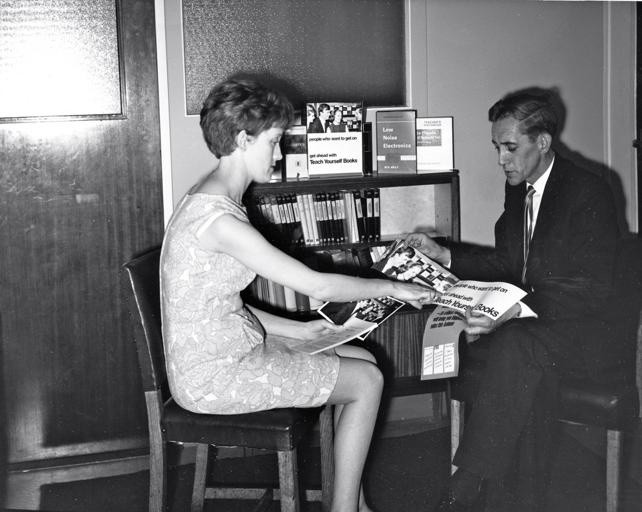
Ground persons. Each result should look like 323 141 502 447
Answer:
158 79 446 512
402 94 639 512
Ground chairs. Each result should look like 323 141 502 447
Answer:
118 245 337 511
448 234 641 512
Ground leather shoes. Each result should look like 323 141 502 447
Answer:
434 471 487 512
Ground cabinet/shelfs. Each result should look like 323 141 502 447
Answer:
243 170 464 399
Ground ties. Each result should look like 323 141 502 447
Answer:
521 186 536 283
